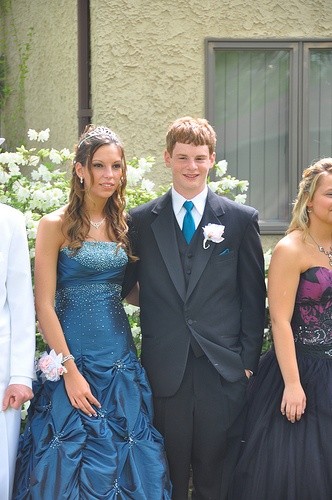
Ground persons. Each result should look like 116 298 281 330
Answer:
309 231 332 267
0 202 36 500
10 124 173 500
121 116 267 499
233 157 331 500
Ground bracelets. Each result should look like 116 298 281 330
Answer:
61 355 74 363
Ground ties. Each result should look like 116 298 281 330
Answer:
182 201 195 245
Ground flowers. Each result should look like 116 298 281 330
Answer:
202 223 226 249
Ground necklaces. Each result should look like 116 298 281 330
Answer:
90 217 106 230
